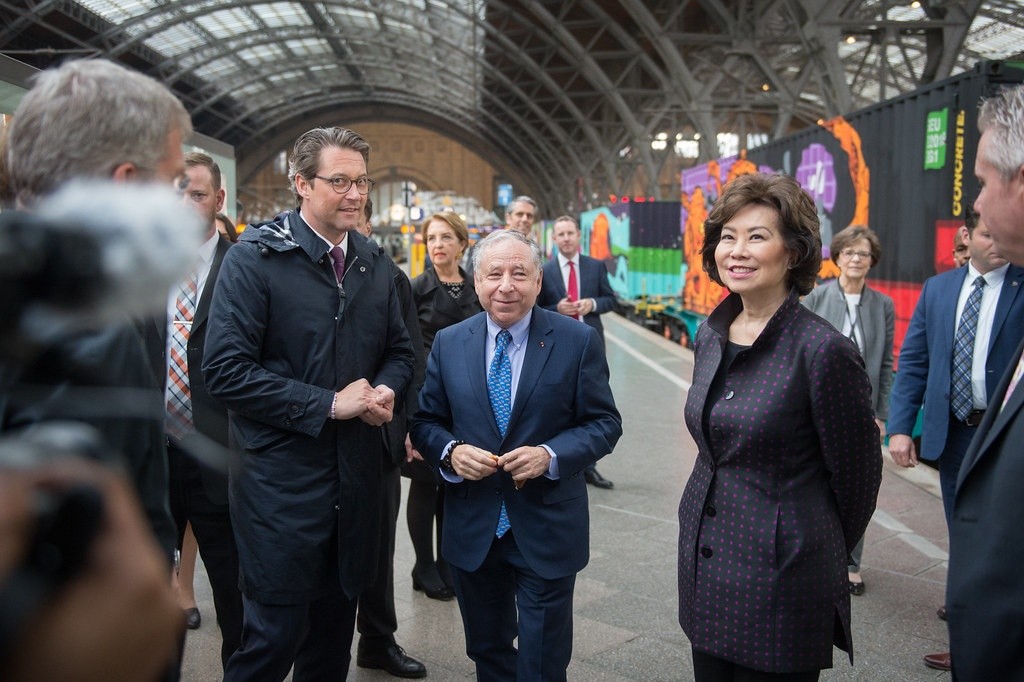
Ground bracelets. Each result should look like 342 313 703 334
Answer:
330 392 337 419
446 440 466 476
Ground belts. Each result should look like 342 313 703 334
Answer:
965 410 984 427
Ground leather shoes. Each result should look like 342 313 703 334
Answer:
924 652 952 671
356 643 428 678
585 469 615 487
849 577 865 596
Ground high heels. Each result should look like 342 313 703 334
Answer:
411 563 458 602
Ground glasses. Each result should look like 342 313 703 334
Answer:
838 248 872 260
314 174 376 196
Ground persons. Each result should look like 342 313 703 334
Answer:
0 57 617 682
678 173 883 682
800 82 1024 682
200 126 417 682
408 229 623 682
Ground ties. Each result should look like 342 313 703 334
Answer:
166 265 197 442
567 261 579 321
331 246 345 283
952 276 986 422
486 330 513 542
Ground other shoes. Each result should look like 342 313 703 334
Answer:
936 605 947 620
184 607 200 629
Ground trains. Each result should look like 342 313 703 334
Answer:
530 52 1024 466
